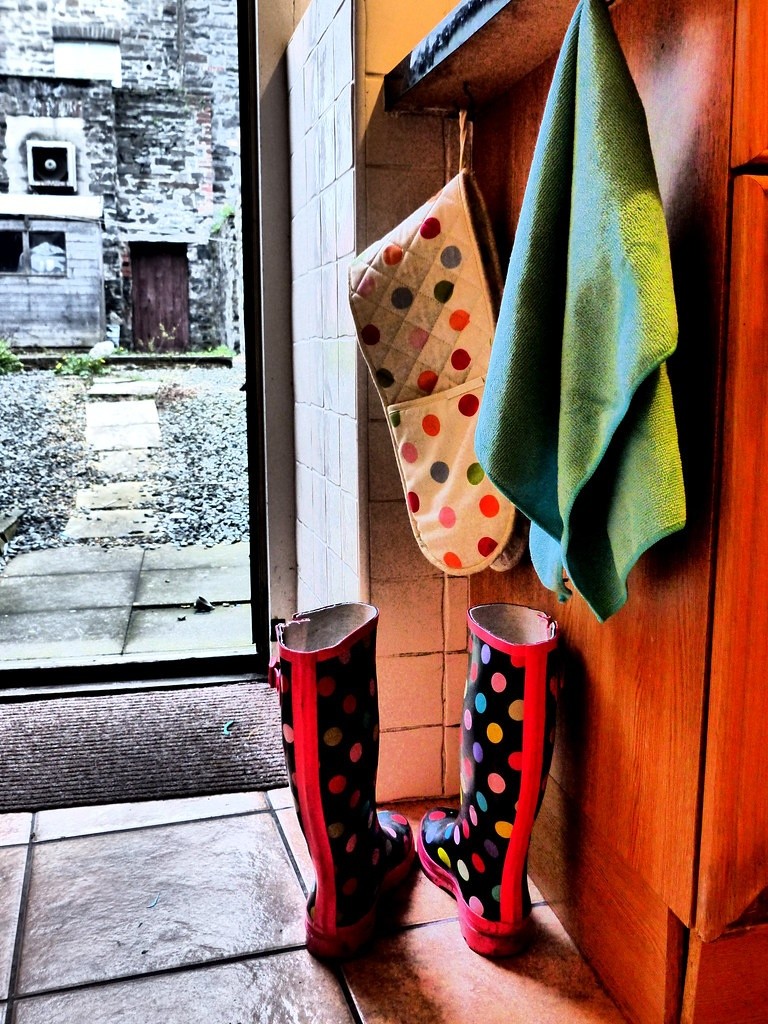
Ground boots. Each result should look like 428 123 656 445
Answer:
418 601 565 958
269 603 416 959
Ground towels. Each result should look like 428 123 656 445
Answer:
472 0 690 624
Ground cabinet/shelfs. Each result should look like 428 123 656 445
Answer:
385 0 768 1024
